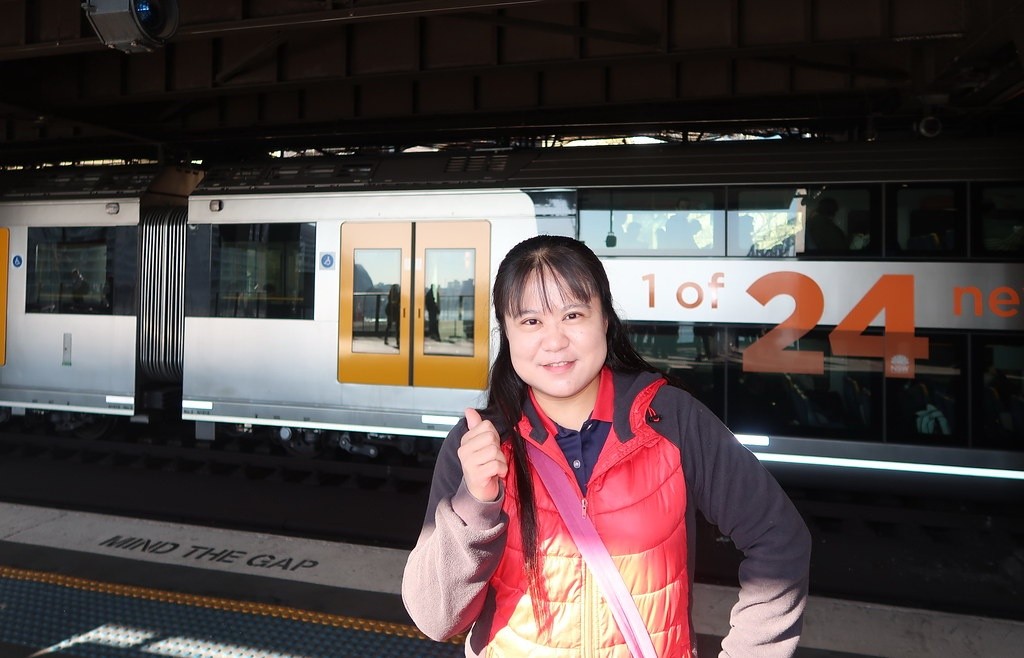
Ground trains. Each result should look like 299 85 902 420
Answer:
0 103 1024 525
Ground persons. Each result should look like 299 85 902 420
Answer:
401 234 811 658
382 284 399 349
426 285 444 342
